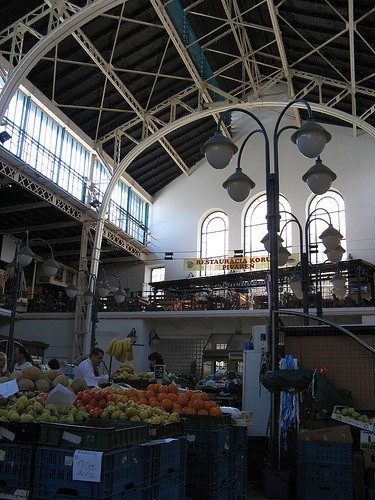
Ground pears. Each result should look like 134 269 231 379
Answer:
0 392 89 423
101 400 180 424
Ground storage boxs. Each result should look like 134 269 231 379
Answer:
298 405 375 500
0 413 247 500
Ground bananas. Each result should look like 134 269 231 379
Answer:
109 338 155 381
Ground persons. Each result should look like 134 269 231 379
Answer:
75 348 104 385
0 352 7 378
12 347 32 373
145 352 168 376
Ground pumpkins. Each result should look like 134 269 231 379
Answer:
0 365 87 394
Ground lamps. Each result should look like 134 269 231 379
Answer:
127 328 137 344
149 330 161 346
0 130 11 143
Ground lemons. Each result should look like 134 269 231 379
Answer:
90 386 126 396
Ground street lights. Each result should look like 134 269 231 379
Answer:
63 268 127 355
260 208 346 327
0 229 60 372
203 96 337 472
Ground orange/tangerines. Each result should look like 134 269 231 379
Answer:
121 384 221 416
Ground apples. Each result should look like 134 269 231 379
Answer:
39 389 121 417
337 407 370 425
